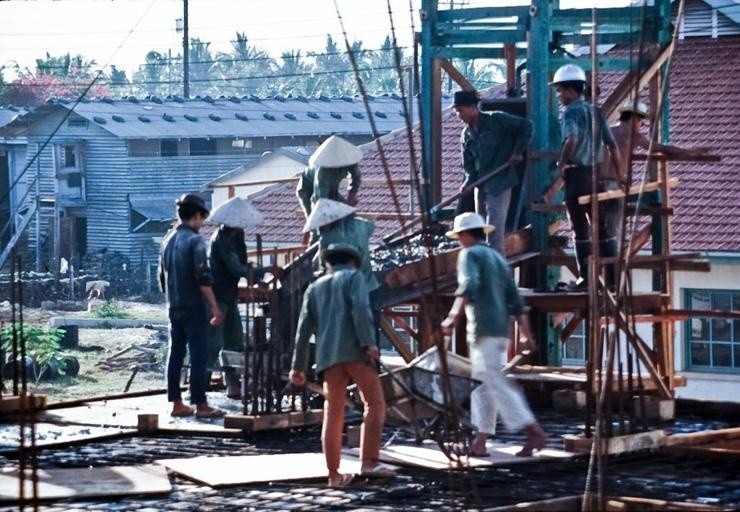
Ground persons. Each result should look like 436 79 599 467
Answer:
454 91 535 254
289 243 396 488
440 211 546 456
302 198 383 374
208 197 283 400
554 65 615 290
296 136 361 248
599 99 692 252
156 193 227 418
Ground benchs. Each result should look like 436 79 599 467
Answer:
45 317 170 349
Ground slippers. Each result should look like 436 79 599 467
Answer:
227 394 242 400
454 444 492 457
196 405 228 418
167 405 196 417
325 469 354 488
511 436 547 458
356 463 401 478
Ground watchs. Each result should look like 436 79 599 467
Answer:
554 161 561 169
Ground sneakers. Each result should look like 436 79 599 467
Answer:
568 277 592 292
603 283 619 292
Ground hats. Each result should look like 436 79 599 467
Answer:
210 196 266 230
307 131 365 168
300 197 358 236
545 62 586 87
174 191 211 220
318 243 364 259
442 212 496 240
618 95 649 118
448 89 483 110
